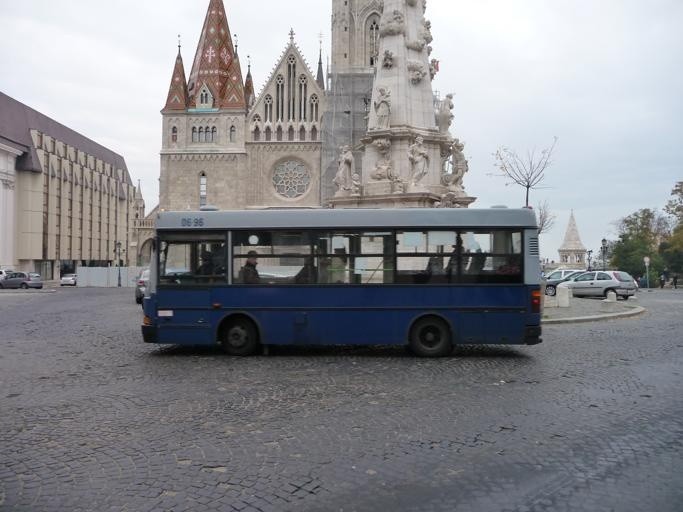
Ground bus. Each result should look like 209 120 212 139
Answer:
139 207 547 361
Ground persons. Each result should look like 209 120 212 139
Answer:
658 273 665 288
672 271 678 289
285 254 317 284
194 250 216 284
326 244 347 284
241 250 276 284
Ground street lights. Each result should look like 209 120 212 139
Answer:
599 238 609 271
586 250 592 271
115 240 123 288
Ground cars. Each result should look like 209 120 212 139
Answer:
542 267 640 301
0 269 76 289
133 267 150 304
